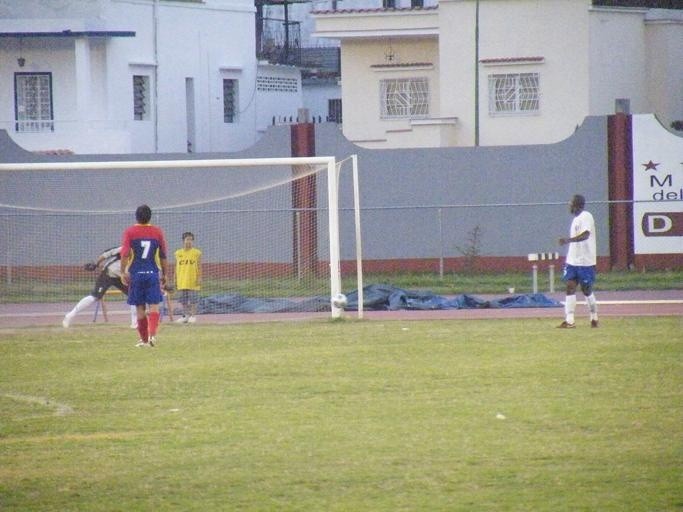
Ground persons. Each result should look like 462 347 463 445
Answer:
555 193 600 330
119 205 168 348
173 233 203 325
63 246 147 330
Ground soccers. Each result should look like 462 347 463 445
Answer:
330 293 348 308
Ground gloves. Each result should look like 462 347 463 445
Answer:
84 264 98 271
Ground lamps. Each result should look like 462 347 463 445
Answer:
16 36 27 67
384 35 395 63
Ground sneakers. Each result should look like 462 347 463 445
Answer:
557 321 576 328
591 320 601 328
